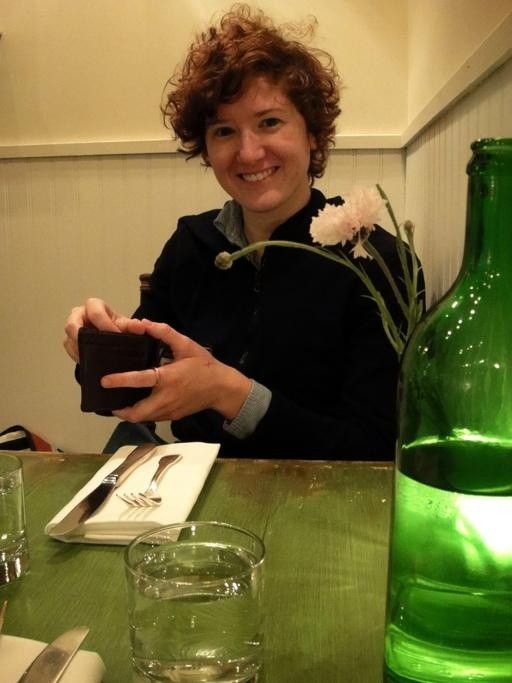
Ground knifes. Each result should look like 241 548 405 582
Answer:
46 443 157 539
19 622 90 683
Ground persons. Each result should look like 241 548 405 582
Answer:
64 4 426 461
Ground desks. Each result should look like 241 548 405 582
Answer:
0 447 395 680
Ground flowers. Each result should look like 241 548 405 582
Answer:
213 175 433 357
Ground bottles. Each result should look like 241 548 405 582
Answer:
383 134 512 683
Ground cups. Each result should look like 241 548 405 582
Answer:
0 448 33 587
122 520 271 683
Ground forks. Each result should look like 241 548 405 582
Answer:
116 450 183 509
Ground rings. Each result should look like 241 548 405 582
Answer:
153 367 160 390
